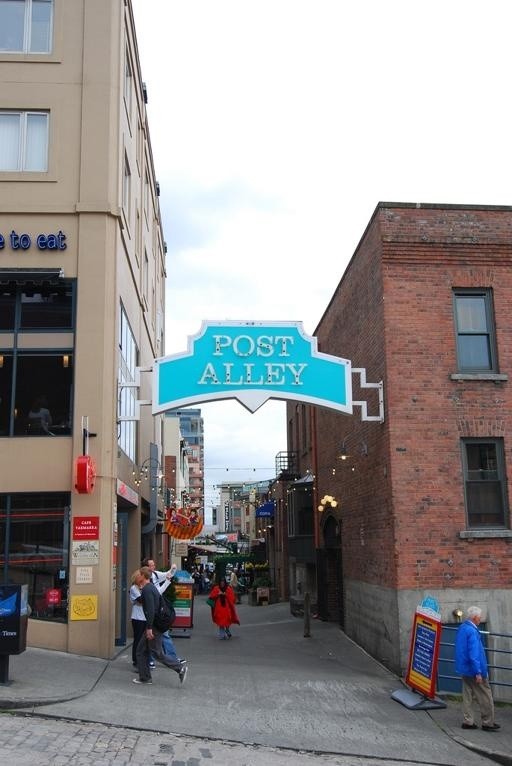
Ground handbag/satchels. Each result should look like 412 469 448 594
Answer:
207 599 214 608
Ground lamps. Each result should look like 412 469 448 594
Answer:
317 495 338 511
339 433 368 460
141 457 166 479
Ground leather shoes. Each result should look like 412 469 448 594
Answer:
462 722 478 729
482 723 500 729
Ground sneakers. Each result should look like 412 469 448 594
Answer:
177 658 187 683
133 659 156 684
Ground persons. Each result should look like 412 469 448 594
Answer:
170 508 199 526
455 606 501 730
129 557 246 685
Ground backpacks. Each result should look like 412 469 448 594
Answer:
148 584 175 632
